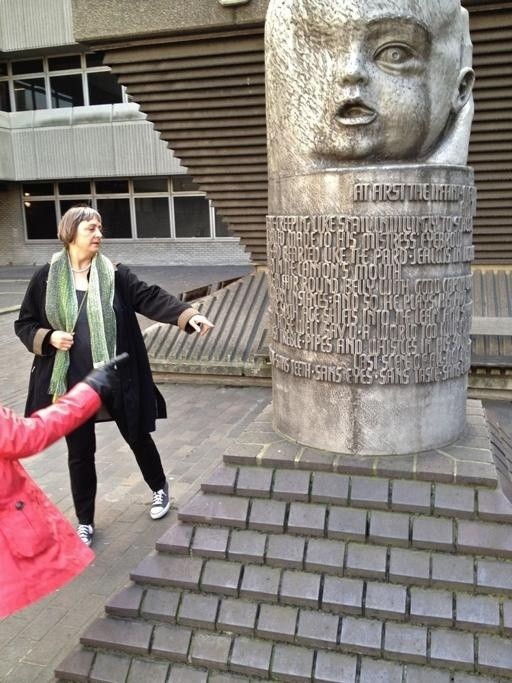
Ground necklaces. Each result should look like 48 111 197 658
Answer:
70 260 91 273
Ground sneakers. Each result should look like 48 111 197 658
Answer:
77 523 96 546
149 482 170 519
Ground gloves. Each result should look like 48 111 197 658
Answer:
85 352 130 400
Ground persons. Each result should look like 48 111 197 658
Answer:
13 205 215 549
0 352 130 620
263 0 477 173
